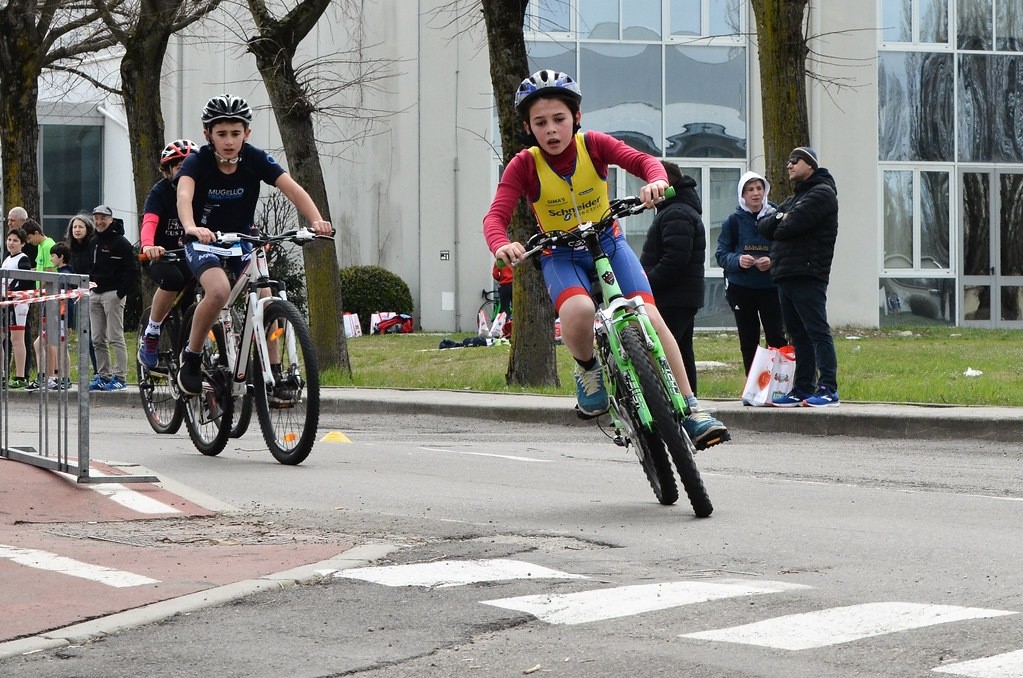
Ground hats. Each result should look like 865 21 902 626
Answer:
789 147 818 172
92 205 113 216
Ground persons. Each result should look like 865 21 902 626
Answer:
484 70 728 446
639 162 706 397
172 94 331 403
757 148 840 407
138 140 200 369
492 263 513 320
0 204 134 392
714 171 789 376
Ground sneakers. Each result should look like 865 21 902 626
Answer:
100 375 128 392
802 385 840 407
90 374 111 390
682 405 728 444
771 391 803 407
176 340 204 395
8 379 29 389
24 380 45 391
51 375 72 390
137 330 160 369
573 364 610 416
266 386 294 403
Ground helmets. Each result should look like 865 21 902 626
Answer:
160 139 200 163
201 94 254 123
514 69 582 114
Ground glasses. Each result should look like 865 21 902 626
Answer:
787 156 801 166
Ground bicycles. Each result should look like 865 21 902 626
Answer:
135 226 337 465
495 183 713 519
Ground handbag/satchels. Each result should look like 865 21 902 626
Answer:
741 345 795 406
342 311 415 338
474 308 509 338
